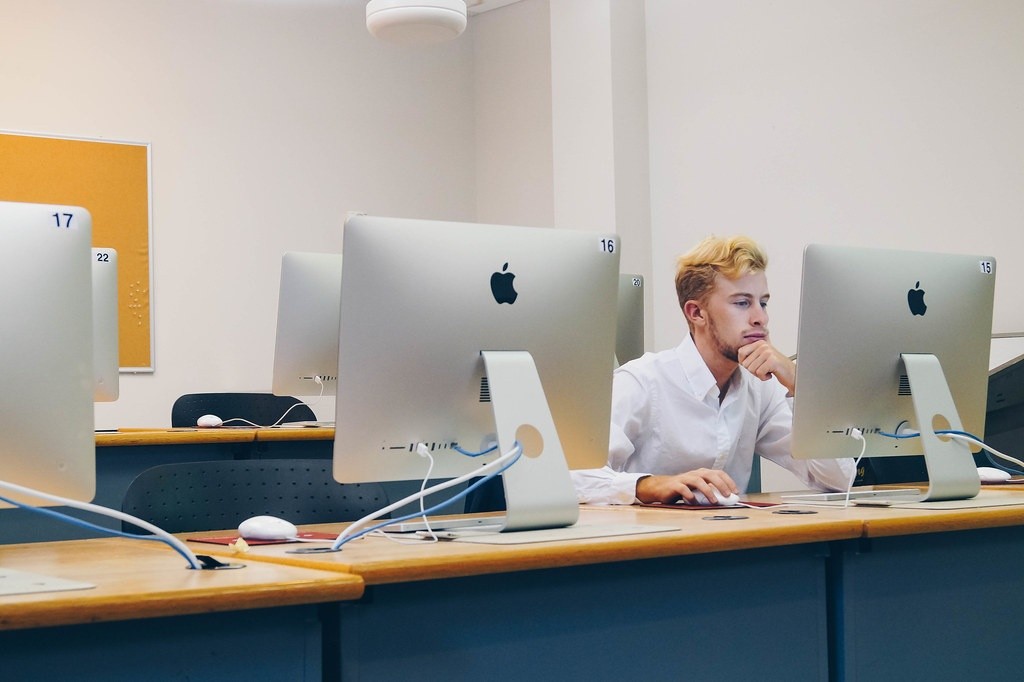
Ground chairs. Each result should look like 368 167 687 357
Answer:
117 458 392 538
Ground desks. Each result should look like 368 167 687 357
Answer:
0 422 1024 682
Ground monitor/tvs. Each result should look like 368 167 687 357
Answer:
272 216 681 543
795 243 1024 509
0 203 122 509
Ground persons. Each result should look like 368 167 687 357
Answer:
570 235 857 507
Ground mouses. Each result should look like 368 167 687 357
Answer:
976 467 1011 483
197 415 223 427
683 482 740 509
238 517 298 539
